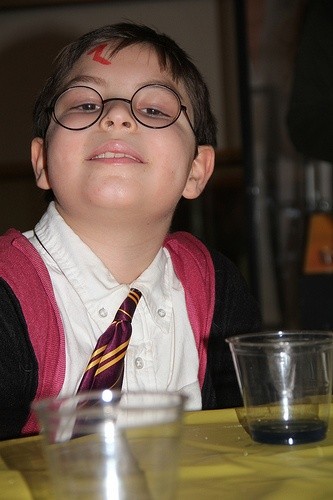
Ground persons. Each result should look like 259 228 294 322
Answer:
0 21 256 445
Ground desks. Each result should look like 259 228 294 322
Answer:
0 406 333 500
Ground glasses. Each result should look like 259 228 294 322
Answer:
43 84 201 146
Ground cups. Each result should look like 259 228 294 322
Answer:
225 329 333 446
30 390 189 499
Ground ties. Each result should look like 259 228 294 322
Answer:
69 287 142 439
